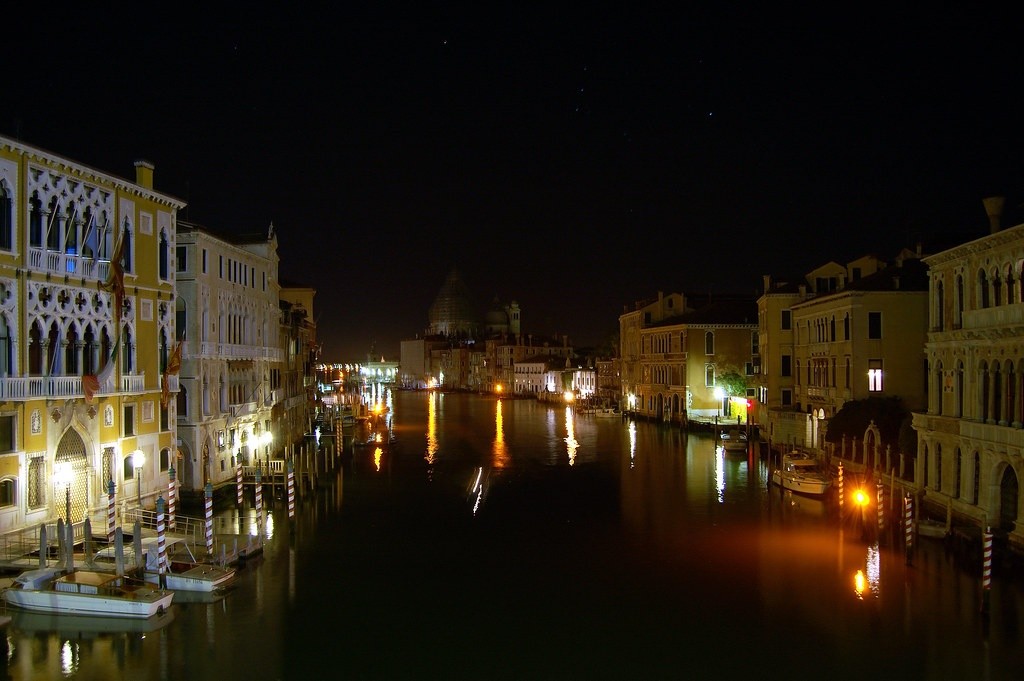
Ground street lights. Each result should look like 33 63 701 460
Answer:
154 496 167 575
254 470 262 532
106 475 115 542
236 452 244 509
287 462 294 520
167 463 176 533
202 483 213 555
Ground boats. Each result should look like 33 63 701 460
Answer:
720 430 748 452
911 519 948 538
769 481 835 515
772 450 829 495
0 602 177 634
581 401 615 414
93 537 238 591
0 568 176 618
595 408 623 418
